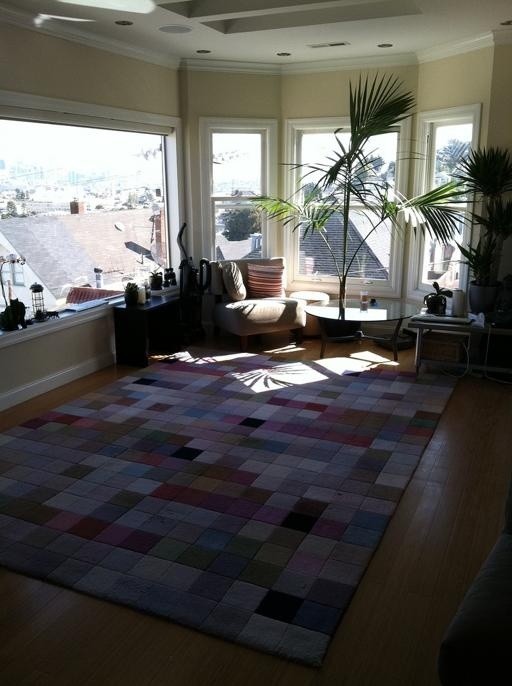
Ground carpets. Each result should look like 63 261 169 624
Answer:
0 345 468 667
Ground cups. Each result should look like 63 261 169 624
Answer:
360 290 370 304
370 299 378 307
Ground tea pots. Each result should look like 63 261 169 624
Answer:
450 288 468 317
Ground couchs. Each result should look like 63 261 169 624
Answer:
200 258 303 352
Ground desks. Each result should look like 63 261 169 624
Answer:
408 307 471 370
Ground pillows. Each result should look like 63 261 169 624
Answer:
247 262 284 298
223 262 246 300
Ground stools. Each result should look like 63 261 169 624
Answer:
290 291 330 336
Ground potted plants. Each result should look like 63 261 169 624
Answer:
423 282 453 314
124 282 139 306
447 145 512 324
149 265 163 290
250 71 482 340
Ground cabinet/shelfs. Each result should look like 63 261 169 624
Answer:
113 288 206 367
